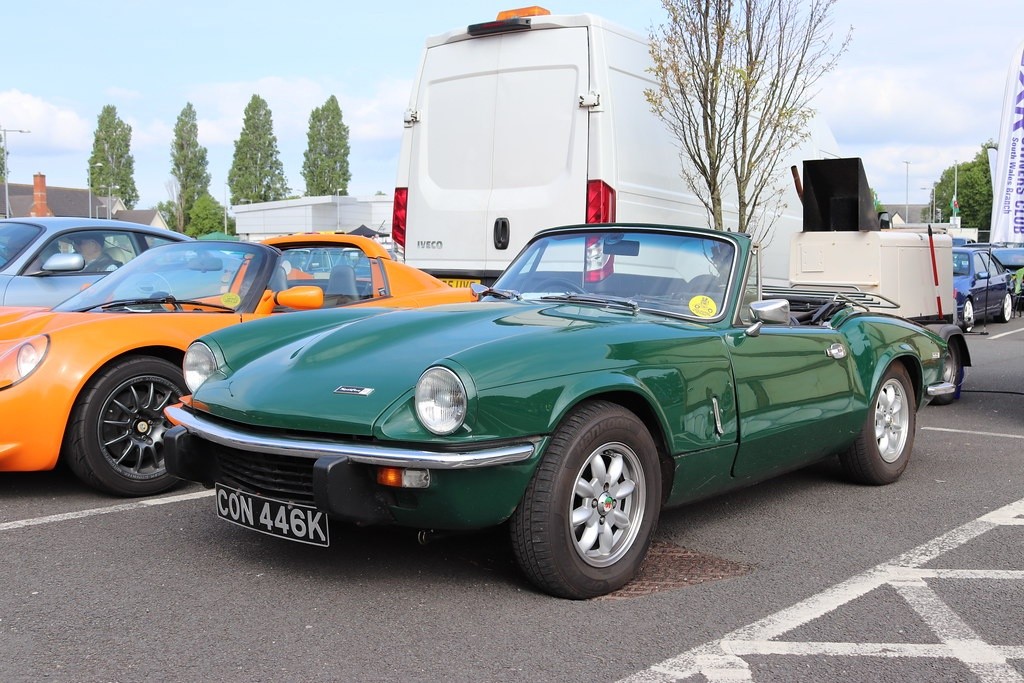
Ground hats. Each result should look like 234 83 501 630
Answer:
68 233 104 247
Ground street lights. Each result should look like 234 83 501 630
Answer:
920 187 936 223
2 129 31 218
88 162 103 218
98 184 119 220
902 160 912 222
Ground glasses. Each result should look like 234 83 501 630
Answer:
71 238 82 245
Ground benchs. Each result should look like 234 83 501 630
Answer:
284 278 374 305
741 295 844 327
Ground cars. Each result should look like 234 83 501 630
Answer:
0 217 245 311
950 235 1024 310
162 223 949 600
951 247 1015 333
0 231 480 500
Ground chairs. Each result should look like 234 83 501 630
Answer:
316 265 364 306
264 267 290 295
667 275 726 308
105 247 128 267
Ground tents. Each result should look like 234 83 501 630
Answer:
346 224 390 244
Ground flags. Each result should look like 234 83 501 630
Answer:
949 195 960 213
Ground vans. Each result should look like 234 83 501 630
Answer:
390 8 889 296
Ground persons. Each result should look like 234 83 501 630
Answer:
73 233 124 272
6 226 42 275
